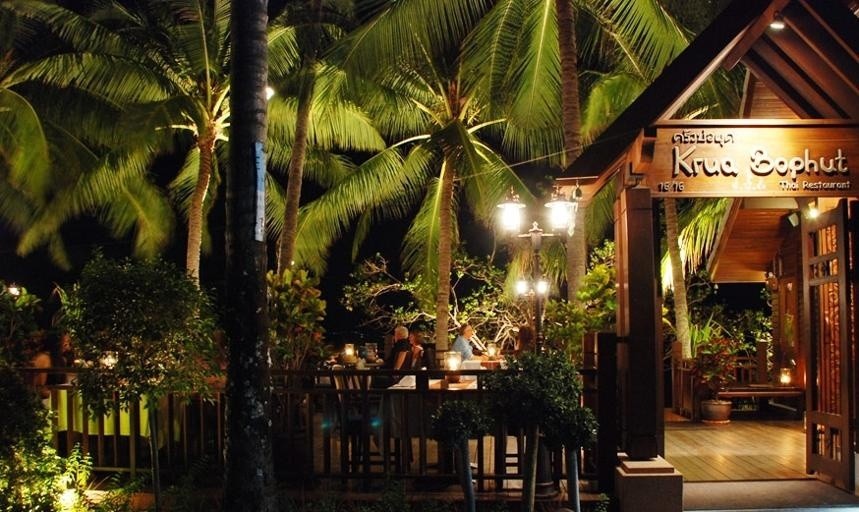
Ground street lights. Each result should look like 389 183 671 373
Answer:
492 178 580 367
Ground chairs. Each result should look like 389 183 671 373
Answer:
328 342 456 484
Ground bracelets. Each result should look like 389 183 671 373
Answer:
394 367 399 371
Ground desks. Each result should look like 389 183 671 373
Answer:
385 373 492 491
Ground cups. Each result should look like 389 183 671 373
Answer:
440 380 449 390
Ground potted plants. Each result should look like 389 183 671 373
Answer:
674 321 760 429
435 344 597 500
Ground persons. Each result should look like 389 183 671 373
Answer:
374 326 413 390
32 325 74 407
499 326 535 358
301 330 326 391
451 323 489 362
408 330 427 369
